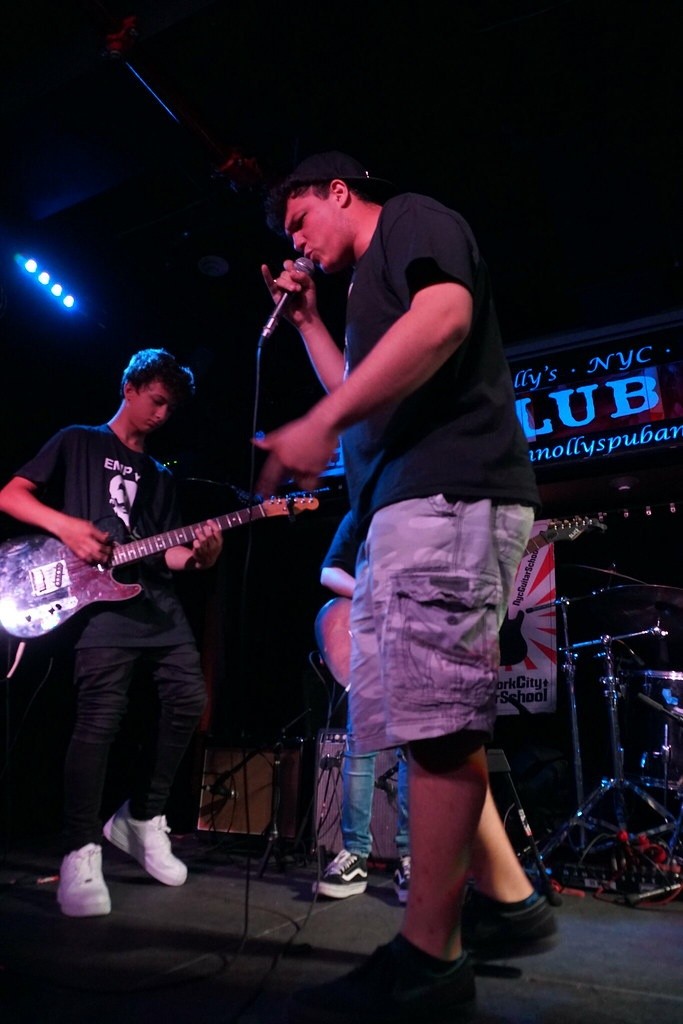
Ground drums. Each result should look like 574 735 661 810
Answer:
631 667 683 793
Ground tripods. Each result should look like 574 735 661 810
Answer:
524 577 683 908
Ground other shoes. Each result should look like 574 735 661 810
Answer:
458 884 559 962
300 944 476 1024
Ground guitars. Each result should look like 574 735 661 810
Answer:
0 491 323 640
316 514 608 694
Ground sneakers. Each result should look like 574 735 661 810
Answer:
103 800 187 886
311 849 368 898
57 842 111 917
393 855 414 902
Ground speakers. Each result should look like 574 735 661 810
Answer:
316 729 402 869
194 732 317 856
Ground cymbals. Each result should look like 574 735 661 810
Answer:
575 565 645 585
588 584 682 635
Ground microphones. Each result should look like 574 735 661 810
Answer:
258 256 315 348
202 785 240 800
373 778 399 797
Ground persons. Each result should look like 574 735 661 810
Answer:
0 348 223 916
250 150 559 1024
311 508 410 903
